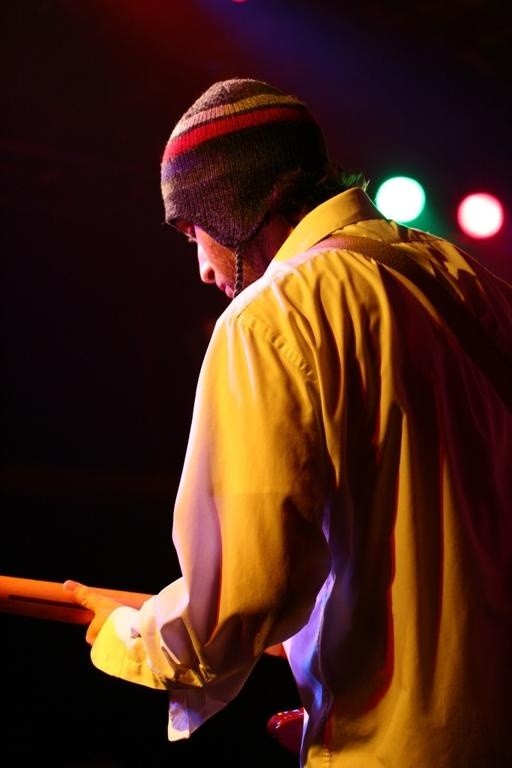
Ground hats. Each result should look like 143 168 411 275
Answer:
160 77 327 247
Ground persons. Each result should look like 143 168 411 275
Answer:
60 77 512 767
